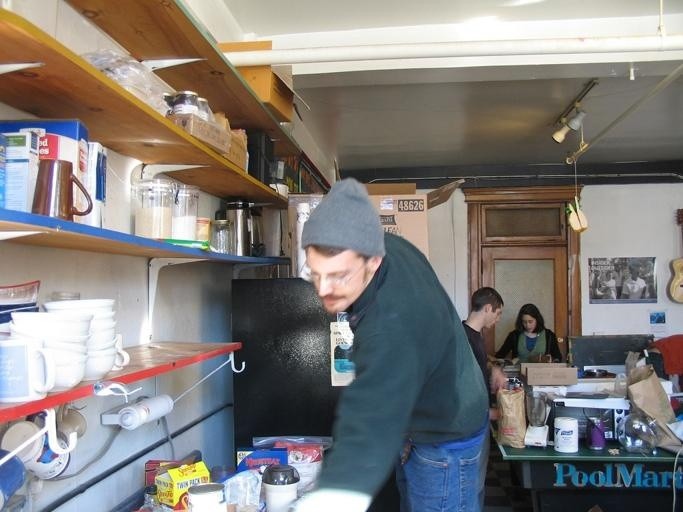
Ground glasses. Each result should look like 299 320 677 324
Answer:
300 259 365 285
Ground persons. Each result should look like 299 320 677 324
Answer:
592 270 617 299
620 262 650 299
495 304 562 363
292 178 489 512
461 287 505 421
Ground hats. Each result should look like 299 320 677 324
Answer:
301 178 385 257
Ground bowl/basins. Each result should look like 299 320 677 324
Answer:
12 299 115 389
262 465 300 485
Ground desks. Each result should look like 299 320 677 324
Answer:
487 420 683 512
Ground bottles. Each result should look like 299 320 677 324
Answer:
211 217 233 254
144 485 159 511
130 177 201 241
585 415 605 450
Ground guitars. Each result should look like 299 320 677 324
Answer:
667 209 683 304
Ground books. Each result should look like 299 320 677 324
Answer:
1 119 107 229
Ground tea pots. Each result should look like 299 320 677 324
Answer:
215 201 265 256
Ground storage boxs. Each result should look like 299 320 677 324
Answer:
216 40 310 121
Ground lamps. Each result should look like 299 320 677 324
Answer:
551 77 599 144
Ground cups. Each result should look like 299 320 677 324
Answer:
264 483 297 512
1 422 70 482
31 159 93 222
113 334 130 373
0 339 55 403
526 391 549 428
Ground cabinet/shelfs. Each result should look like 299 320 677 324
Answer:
0 0 291 424
